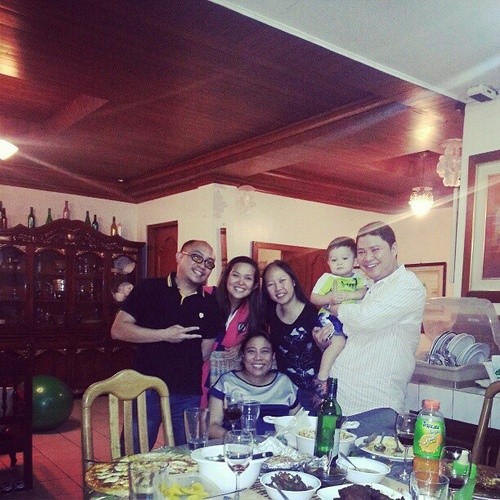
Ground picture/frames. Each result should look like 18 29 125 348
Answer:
404 262 447 333
462 150 500 301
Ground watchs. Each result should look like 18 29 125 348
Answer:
325 304 331 310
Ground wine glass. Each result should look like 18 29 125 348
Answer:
395 413 417 480
243 399 259 439
223 393 245 434
224 430 253 500
439 446 473 500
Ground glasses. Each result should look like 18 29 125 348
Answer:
181 250 216 270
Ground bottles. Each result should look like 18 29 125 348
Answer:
314 377 343 458
62 200 70 219
84 210 98 230
111 216 118 236
27 206 36 228
413 400 446 492
46 207 53 223
0 201 8 229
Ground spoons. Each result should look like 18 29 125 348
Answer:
375 431 387 453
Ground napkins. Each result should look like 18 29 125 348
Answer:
476 354 500 387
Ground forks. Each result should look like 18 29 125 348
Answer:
394 435 403 453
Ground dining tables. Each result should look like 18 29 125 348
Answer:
91 433 413 500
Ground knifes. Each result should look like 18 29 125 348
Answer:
355 431 380 450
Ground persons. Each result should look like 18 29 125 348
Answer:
207 330 302 440
110 240 225 457
201 256 261 438
312 221 427 439
262 260 321 416
309 236 368 400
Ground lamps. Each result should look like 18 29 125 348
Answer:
436 138 462 187
408 150 434 217
237 181 256 211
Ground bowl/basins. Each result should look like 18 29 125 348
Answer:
335 457 391 483
155 472 226 500
291 428 357 458
262 415 360 450
191 444 262 495
260 470 322 500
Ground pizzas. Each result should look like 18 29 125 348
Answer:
84 453 198 497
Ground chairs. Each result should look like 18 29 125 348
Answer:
80 369 175 500
0 344 34 490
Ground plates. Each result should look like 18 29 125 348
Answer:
317 481 404 500
428 330 490 365
356 435 415 462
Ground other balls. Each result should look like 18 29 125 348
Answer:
18 373 74 434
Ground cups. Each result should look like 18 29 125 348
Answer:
129 456 169 500
408 470 450 500
184 408 212 451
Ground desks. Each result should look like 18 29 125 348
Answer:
406 383 500 429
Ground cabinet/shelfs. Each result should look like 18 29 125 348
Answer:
0 219 145 402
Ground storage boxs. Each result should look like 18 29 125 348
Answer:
413 296 500 390
